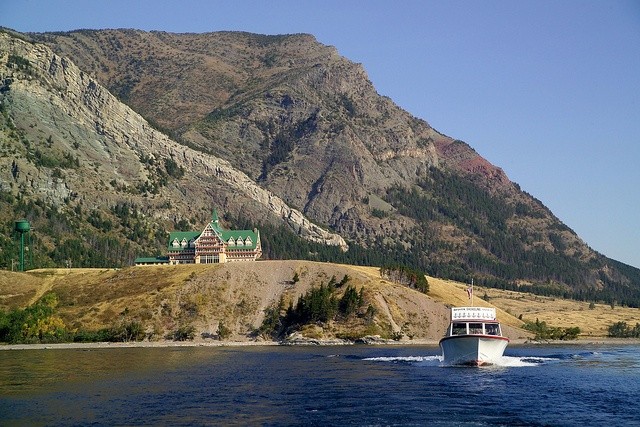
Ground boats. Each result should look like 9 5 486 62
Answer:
440 307 509 367
